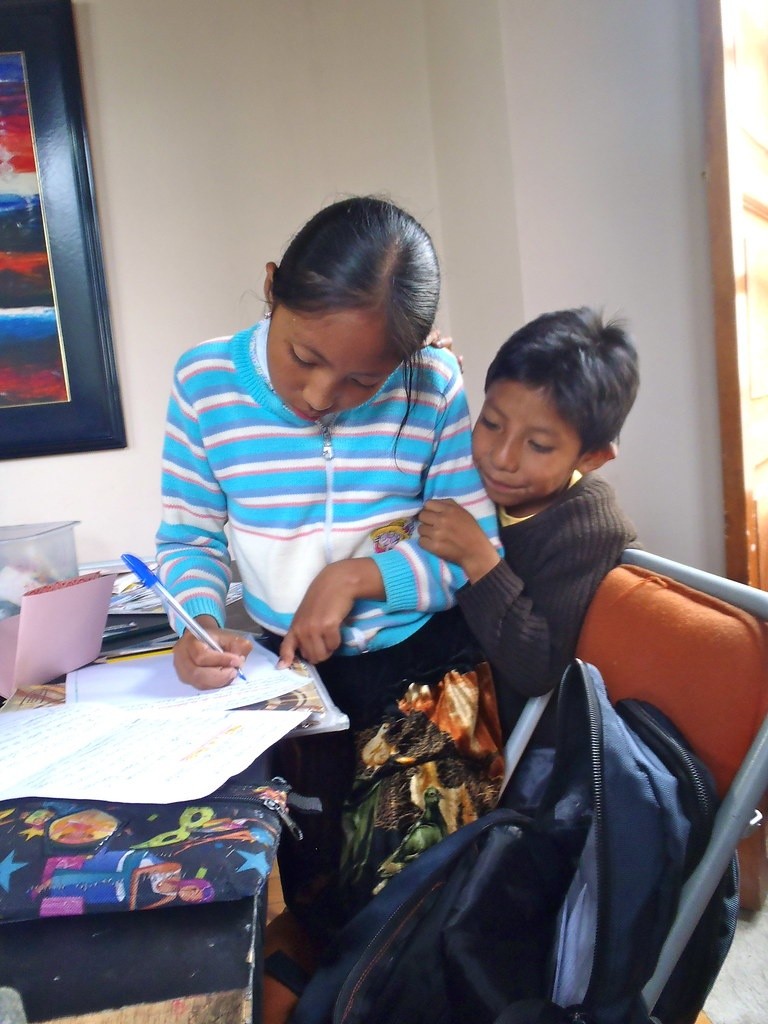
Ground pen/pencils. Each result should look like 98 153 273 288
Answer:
120 552 251 684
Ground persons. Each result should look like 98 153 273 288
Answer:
158 196 505 966
417 297 639 740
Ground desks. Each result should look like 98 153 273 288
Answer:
0 554 272 1024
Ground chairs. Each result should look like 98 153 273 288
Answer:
256 548 768 1024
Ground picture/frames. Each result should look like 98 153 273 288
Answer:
0 0 129 460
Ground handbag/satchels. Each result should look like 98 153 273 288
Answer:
2 773 326 925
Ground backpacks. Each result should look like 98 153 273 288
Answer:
287 657 741 1024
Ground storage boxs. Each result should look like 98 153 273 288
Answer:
1 520 81 620
